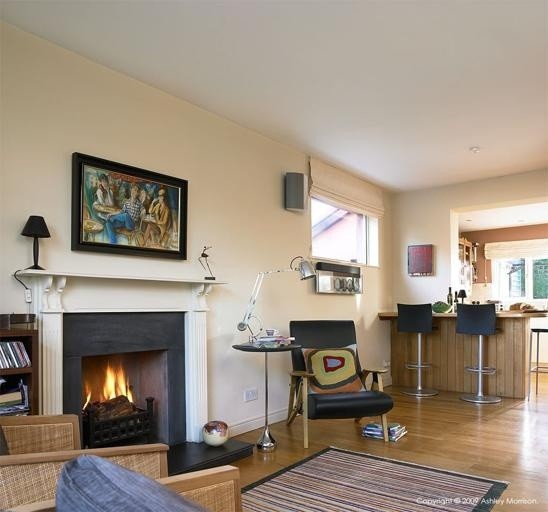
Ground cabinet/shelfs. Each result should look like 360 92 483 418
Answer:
0 314 39 416
459 237 472 295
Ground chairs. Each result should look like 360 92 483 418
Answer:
3 454 242 512
286 320 393 449
455 302 502 404
397 303 440 397
527 329 548 402
0 414 170 512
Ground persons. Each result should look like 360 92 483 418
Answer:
93 173 173 247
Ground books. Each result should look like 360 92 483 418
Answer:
0 341 32 416
360 423 408 443
259 336 295 341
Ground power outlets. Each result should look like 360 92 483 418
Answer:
243 389 260 404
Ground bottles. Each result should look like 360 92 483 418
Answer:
454 291 458 312
447 287 452 313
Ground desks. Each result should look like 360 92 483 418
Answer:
233 343 302 449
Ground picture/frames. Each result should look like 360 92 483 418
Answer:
71 152 188 260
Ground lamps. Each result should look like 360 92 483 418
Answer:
20 215 51 269
457 290 467 304
238 256 317 341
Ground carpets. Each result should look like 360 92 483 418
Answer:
241 446 510 512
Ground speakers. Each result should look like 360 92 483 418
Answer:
285 173 309 211
